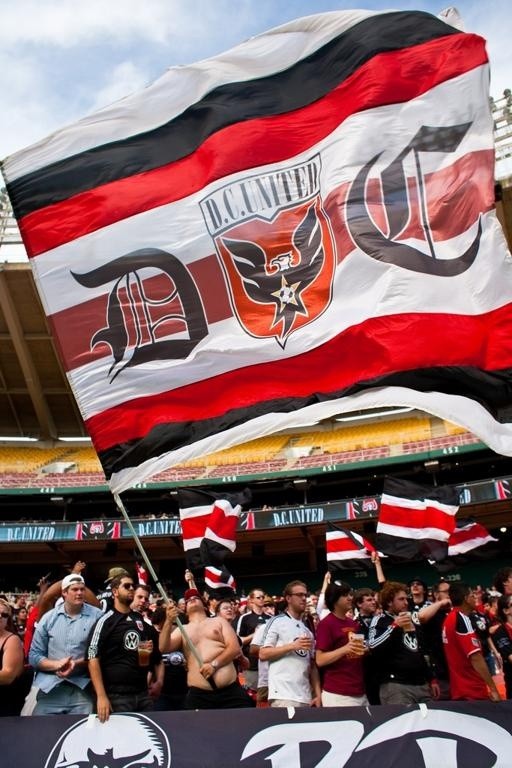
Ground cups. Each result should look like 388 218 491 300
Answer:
350 633 364 659
299 633 310 653
399 610 412 633
137 642 151 668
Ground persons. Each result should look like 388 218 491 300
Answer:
1 503 269 526
1 561 274 721
260 571 512 707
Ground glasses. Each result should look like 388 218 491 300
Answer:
264 603 273 607
1 613 9 617
124 583 136 589
289 593 309 597
255 596 264 599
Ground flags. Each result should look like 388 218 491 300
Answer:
375 472 457 562
2 7 512 494
422 515 500 566
173 500 242 570
324 518 391 574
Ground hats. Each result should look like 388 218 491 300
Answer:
184 589 198 600
104 567 128 583
407 576 423 585
61 574 85 589
